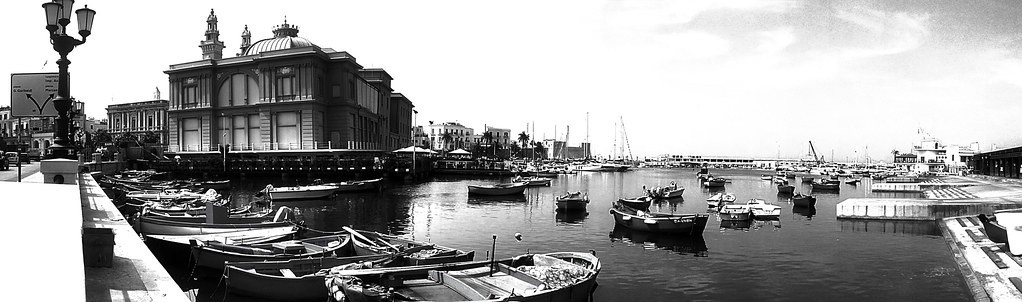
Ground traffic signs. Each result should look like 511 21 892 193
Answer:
10 73 70 117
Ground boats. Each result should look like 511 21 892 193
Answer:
761 161 922 194
98 168 308 248
696 166 782 221
609 196 709 235
640 181 685 199
609 223 709 259
556 190 590 212
790 191 817 207
257 177 385 199
468 177 552 196
190 224 603 302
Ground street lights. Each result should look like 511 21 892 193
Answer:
40 0 96 183
429 120 434 156
413 109 418 177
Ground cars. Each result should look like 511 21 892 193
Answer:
0 151 30 170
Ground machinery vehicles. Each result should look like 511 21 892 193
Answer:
808 141 824 162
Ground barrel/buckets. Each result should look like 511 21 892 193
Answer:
327 240 340 256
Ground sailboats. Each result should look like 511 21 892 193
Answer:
513 111 631 177
